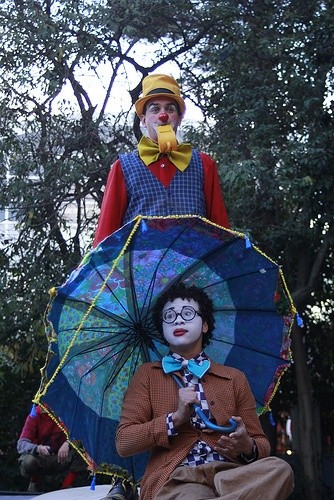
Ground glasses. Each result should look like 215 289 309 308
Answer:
158 305 205 324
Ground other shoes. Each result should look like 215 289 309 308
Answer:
101 477 138 499
25 480 40 494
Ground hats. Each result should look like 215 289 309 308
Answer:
133 74 186 120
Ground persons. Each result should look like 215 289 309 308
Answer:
94 73 231 500
114 283 295 500
17 401 79 494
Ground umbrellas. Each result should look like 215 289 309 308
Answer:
32 214 298 491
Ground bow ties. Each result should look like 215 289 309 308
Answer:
162 355 211 380
137 134 194 173
